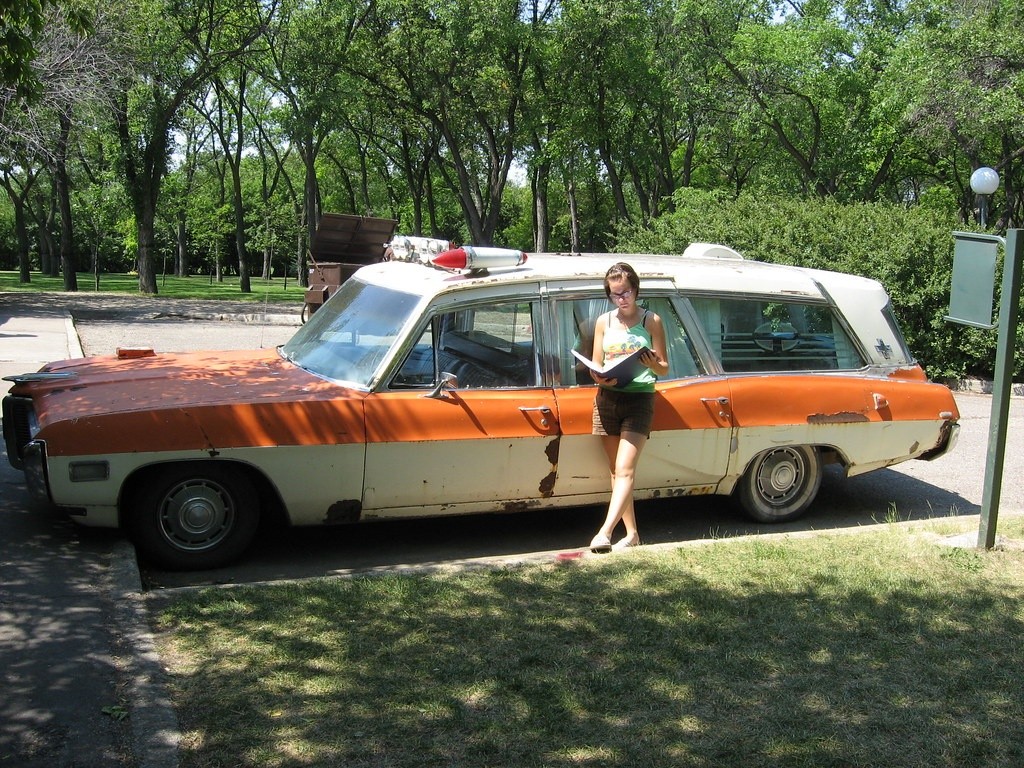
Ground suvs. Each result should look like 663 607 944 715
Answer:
1 240 963 571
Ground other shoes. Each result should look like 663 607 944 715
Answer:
590 535 612 549
613 538 640 551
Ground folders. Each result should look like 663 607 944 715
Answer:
566 346 662 390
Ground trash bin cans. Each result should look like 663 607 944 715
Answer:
305 212 400 319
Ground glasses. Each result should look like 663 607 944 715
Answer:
609 289 635 301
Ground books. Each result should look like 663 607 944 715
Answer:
570 346 661 390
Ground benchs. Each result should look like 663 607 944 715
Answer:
413 350 517 388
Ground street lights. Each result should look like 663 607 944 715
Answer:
969 166 999 232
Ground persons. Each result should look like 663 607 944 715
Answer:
590 261 669 552
